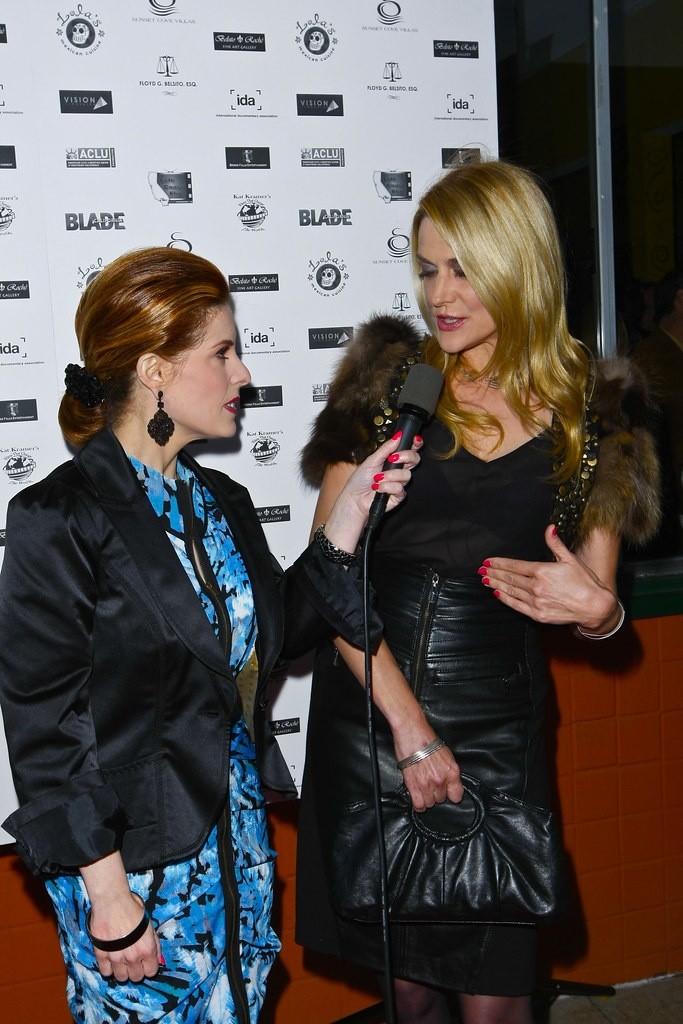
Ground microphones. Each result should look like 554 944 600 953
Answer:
368 363 446 530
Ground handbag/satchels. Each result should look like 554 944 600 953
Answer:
330 772 559 924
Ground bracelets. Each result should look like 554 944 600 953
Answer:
314 522 363 568
574 602 626 640
85 890 149 953
395 736 447 770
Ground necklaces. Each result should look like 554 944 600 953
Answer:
458 363 527 390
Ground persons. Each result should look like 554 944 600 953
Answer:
0 247 427 1024
305 161 661 1024
